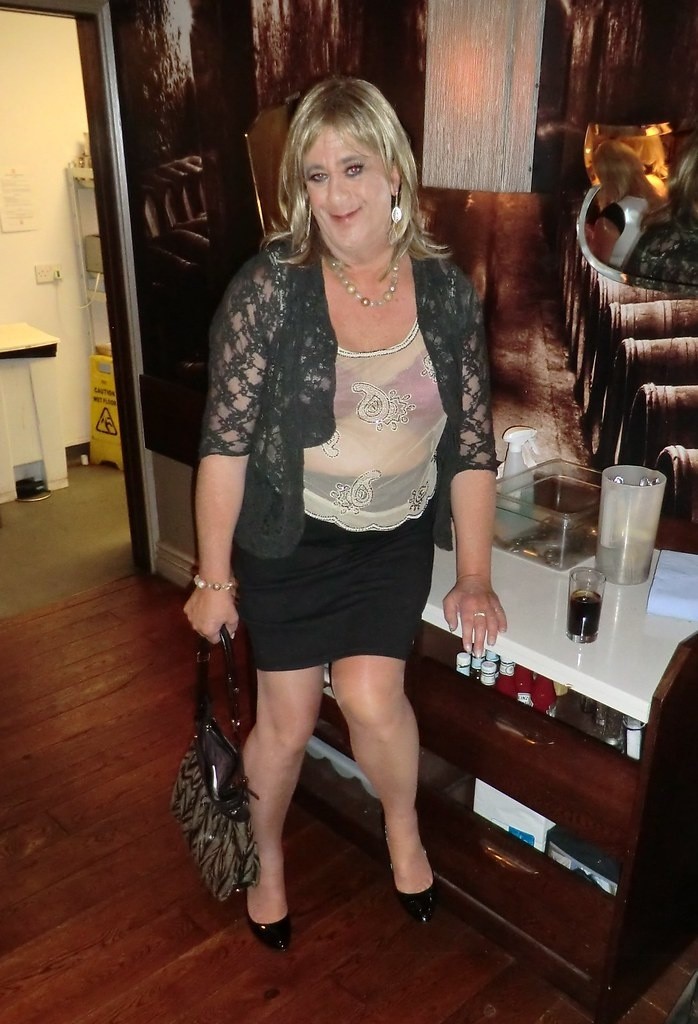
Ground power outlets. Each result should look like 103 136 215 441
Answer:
33 263 64 283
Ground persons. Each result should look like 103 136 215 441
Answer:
182 76 509 950
590 130 698 286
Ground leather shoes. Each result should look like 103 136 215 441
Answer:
246 902 291 949
382 809 439 924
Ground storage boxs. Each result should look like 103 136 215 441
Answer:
472 778 555 852
545 823 621 897
492 456 604 569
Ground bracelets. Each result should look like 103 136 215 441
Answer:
194 575 235 591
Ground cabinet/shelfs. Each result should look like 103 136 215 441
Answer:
65 161 112 355
244 537 698 1024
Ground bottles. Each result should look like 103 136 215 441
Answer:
456 648 645 759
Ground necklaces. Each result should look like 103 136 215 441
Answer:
323 255 401 306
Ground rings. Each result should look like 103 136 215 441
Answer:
473 612 484 617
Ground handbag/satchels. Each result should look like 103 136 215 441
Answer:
170 624 260 901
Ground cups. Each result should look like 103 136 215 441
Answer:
568 568 606 644
596 464 666 586
